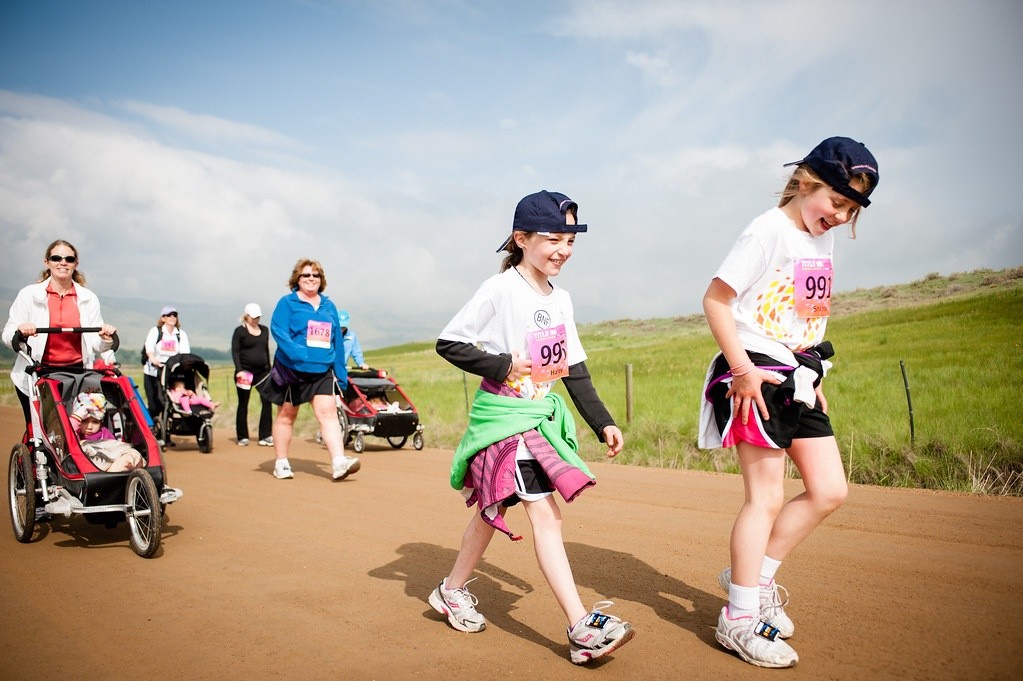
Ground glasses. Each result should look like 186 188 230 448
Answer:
300 273 320 278
165 313 178 317
47 255 76 263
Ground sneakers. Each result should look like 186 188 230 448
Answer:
333 455 361 480
714 606 798 668
719 566 795 640
566 601 636 664
272 458 294 479
427 577 487 632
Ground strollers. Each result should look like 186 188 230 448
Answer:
335 366 425 452
153 354 214 454
9 326 170 558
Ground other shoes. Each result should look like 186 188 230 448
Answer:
258 435 274 446
237 438 250 446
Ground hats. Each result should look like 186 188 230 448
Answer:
783 136 880 207
496 189 588 254
71 392 108 420
244 303 262 319
161 305 177 316
336 310 350 329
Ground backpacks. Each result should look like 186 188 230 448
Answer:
140 325 180 366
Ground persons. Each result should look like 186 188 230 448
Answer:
69 387 144 473
232 302 274 447
171 380 220 415
270 258 361 480
367 395 400 412
698 136 880 668
428 190 636 664
315 309 368 445
2 241 117 521
143 306 191 447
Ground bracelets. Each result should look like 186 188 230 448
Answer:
506 360 514 378
727 360 754 376
